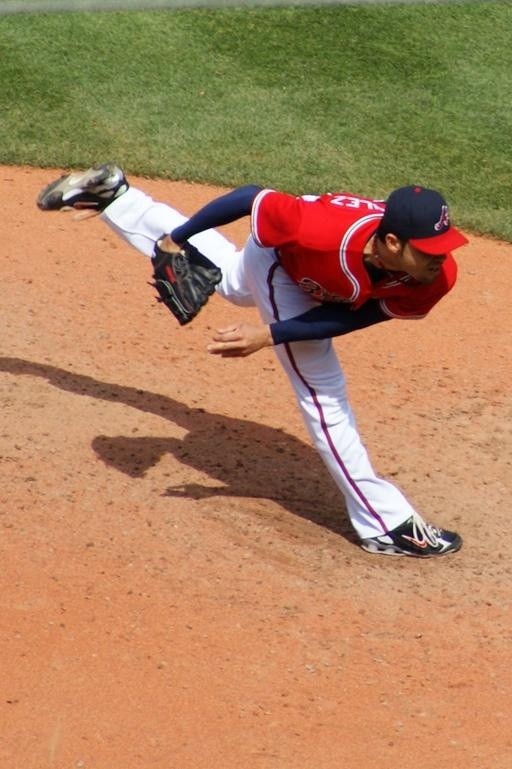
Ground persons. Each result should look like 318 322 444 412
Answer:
35 163 470 560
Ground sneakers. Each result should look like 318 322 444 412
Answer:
37 165 129 211
360 513 463 558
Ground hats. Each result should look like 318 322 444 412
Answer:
384 185 469 257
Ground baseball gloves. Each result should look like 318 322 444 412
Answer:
147 235 222 326
